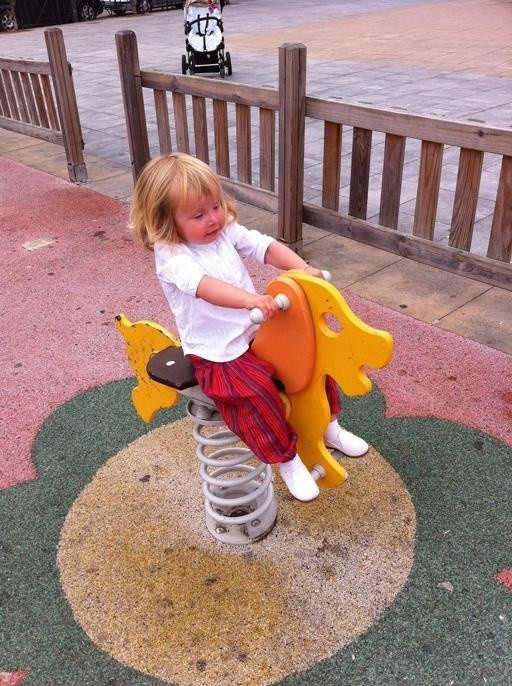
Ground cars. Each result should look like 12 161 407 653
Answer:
102 0 231 15
0 0 104 32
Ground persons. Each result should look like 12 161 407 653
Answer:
129 152 369 502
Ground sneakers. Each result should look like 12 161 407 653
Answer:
279 453 320 502
325 418 369 457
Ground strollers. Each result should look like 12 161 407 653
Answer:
179 1 236 80
